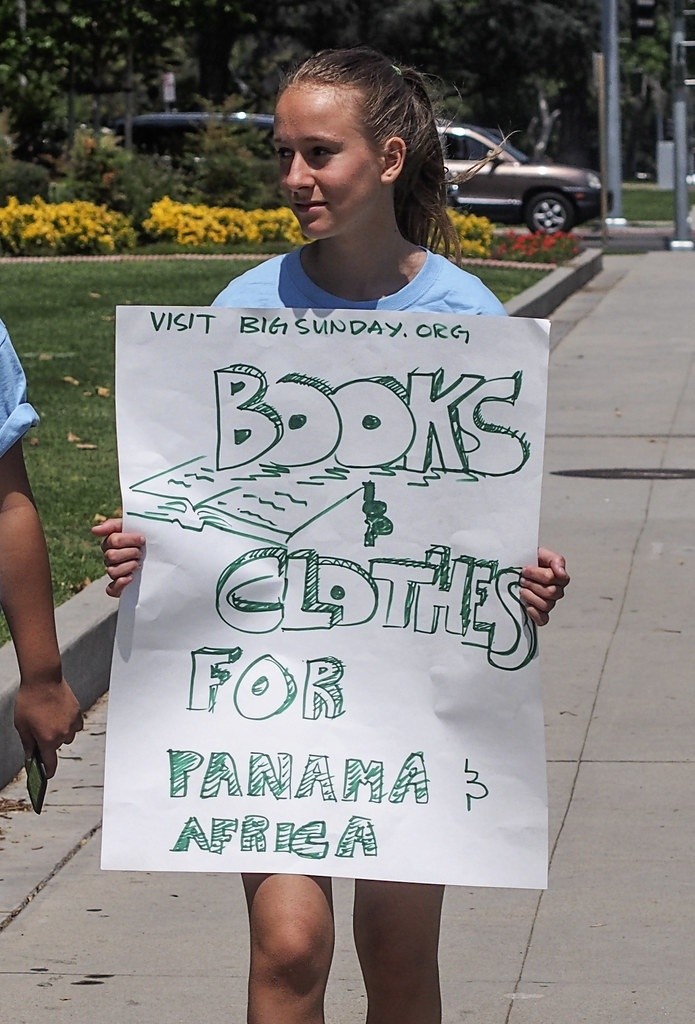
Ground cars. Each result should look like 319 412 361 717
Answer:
435 120 614 235
125 111 276 159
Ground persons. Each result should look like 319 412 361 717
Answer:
2 310 84 771
93 45 575 1024
